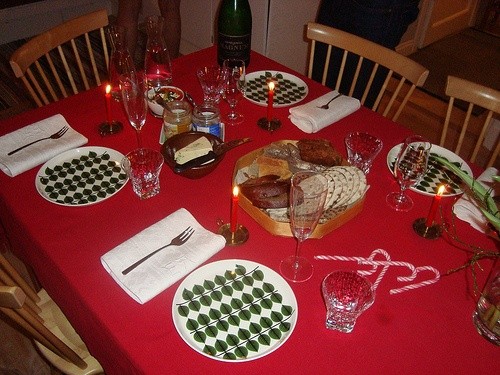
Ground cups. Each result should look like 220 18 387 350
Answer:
345 131 383 175
322 270 376 334
119 147 165 199
472 252 500 346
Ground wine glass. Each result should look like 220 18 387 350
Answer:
385 135 432 212
118 70 148 177
222 58 246 126
197 63 230 123
279 170 328 283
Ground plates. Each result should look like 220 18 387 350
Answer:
235 70 309 108
387 142 474 197
232 139 368 240
170 258 298 363
34 145 133 207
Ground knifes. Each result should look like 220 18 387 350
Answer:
173 136 253 173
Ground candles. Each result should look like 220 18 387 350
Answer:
230 186 240 232
105 85 113 124
267 82 275 121
426 185 445 226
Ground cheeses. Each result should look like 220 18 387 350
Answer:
174 137 213 164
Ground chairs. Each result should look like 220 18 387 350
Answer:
0 10 500 375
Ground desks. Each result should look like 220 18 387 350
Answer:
0 48 500 375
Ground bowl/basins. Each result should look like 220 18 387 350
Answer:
160 131 226 179
145 85 184 118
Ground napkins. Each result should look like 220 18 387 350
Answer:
290 90 361 131
0 114 88 178
453 167 500 233
98 208 227 304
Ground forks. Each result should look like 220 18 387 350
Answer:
121 226 195 276
8 124 69 156
316 92 342 110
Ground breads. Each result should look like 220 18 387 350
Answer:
238 140 367 220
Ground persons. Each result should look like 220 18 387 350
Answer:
116 0 181 65
311 0 421 110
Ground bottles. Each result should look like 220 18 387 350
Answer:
107 26 138 103
162 101 192 139
143 15 173 88
215 0 253 73
191 104 221 141
